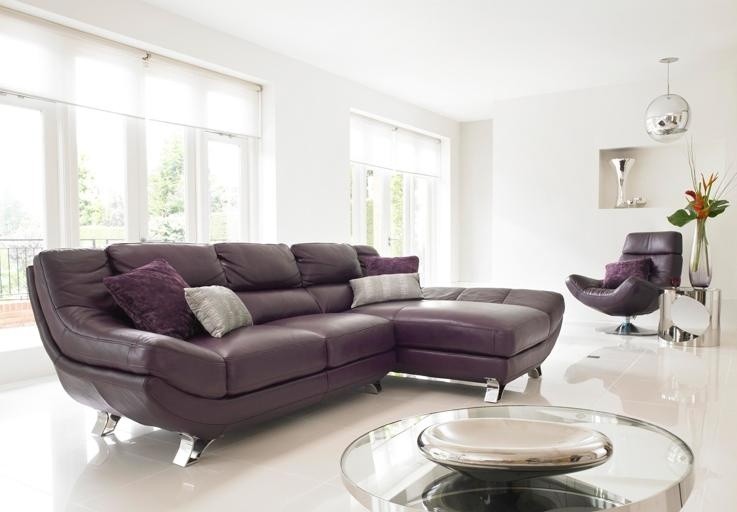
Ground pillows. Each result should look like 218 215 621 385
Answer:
600 257 653 290
181 283 253 339
348 272 425 309
358 254 420 275
100 258 208 339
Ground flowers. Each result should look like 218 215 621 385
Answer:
667 144 734 277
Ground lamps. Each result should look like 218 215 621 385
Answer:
641 55 692 142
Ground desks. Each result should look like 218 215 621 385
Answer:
656 286 723 348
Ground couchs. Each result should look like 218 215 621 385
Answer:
24 238 567 469
564 230 684 339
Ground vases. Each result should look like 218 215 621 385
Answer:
689 215 713 289
